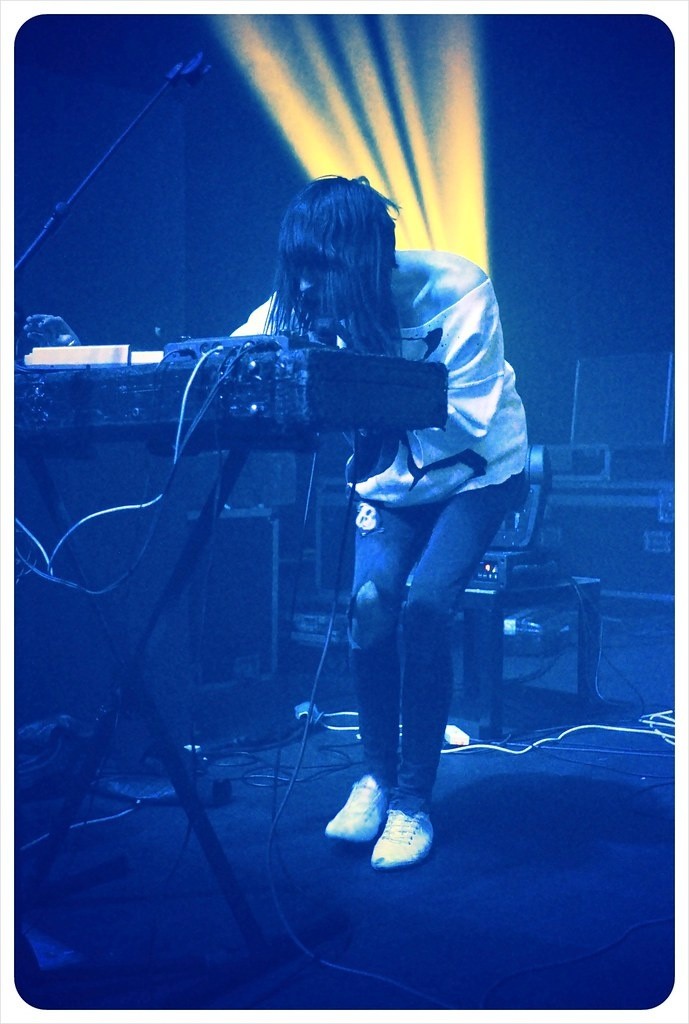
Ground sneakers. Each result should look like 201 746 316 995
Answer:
325 775 388 842
371 809 433 872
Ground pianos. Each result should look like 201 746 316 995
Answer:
14 344 450 434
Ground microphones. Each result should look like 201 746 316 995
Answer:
175 49 215 85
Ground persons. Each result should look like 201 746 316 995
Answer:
230 178 531 869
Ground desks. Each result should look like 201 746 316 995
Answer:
461 577 602 748
